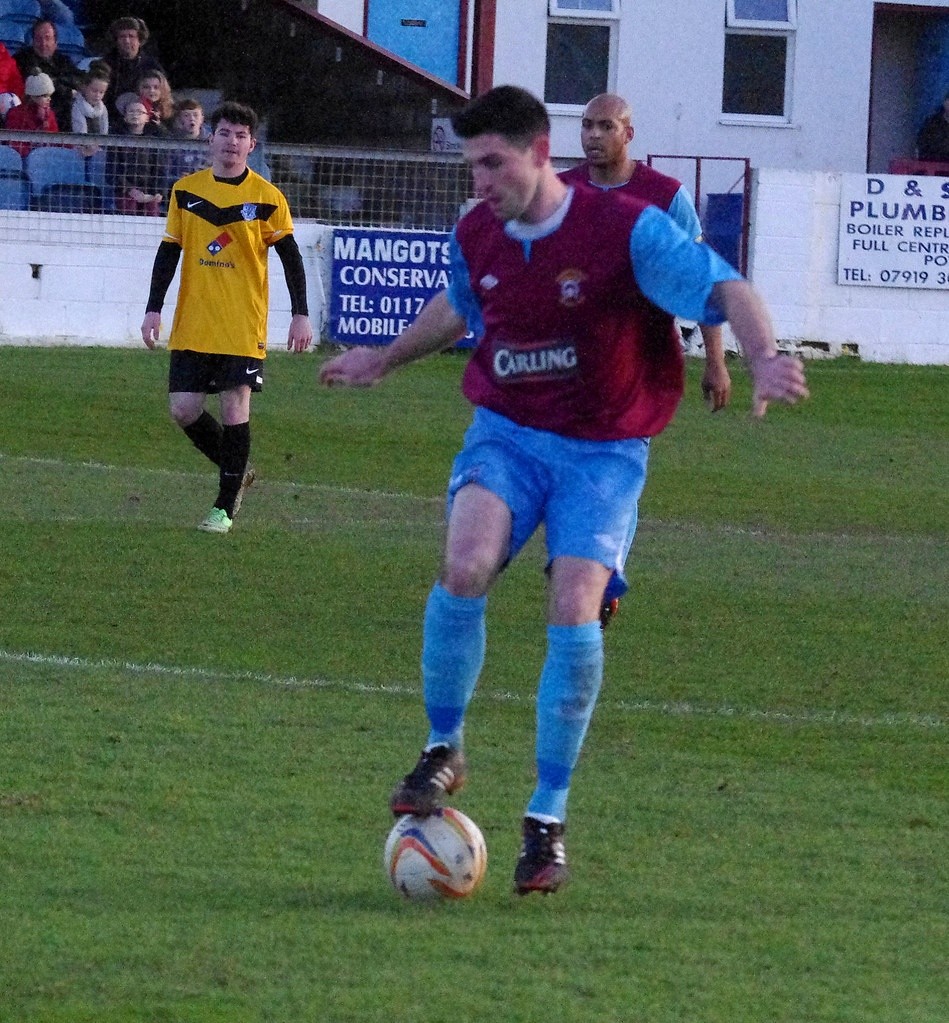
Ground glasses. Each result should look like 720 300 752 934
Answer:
126 107 150 114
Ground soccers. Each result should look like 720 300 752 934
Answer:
383 806 488 901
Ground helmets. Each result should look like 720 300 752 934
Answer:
0 92 22 119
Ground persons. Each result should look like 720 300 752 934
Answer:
316 85 807 897
141 105 312 532
0 0 270 216
557 93 733 631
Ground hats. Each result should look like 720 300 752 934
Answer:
111 16 148 46
24 66 55 96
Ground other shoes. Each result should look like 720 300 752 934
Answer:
599 594 619 629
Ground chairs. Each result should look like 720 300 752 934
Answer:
0 0 364 217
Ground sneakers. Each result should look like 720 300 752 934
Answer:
233 460 254 518
196 507 233 535
389 744 465 818
514 815 567 895
